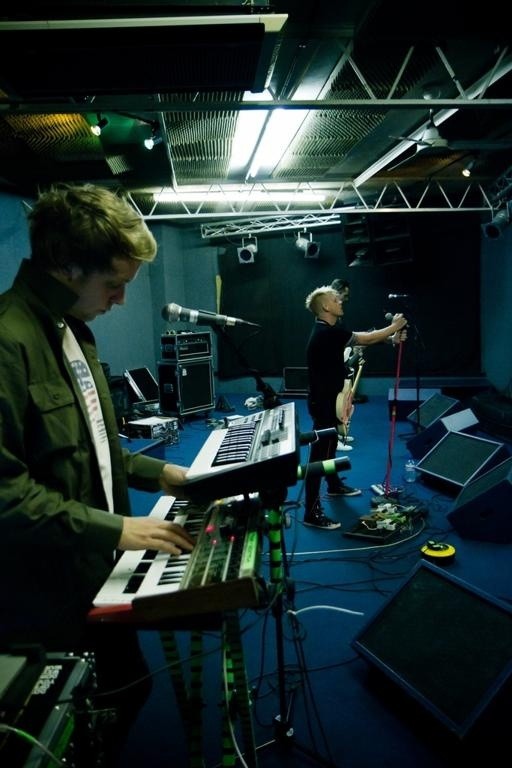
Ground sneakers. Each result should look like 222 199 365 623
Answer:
302 512 342 529
326 484 362 497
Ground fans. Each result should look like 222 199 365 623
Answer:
388 89 511 172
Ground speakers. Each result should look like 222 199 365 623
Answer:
415 429 510 489
349 559 510 740
408 392 465 430
446 456 512 529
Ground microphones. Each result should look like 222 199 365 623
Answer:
384 312 411 328
388 293 410 298
161 301 260 331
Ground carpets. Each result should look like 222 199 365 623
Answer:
118 394 512 767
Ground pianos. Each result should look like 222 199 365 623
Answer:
93 492 263 607
184 401 296 484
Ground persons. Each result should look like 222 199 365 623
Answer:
302 284 408 530
324 277 355 452
0 183 193 756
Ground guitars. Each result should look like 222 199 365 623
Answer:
336 356 365 437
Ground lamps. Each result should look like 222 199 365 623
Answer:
296 238 321 258
462 159 477 177
144 136 163 150
154 192 325 202
91 117 109 136
237 244 258 264
